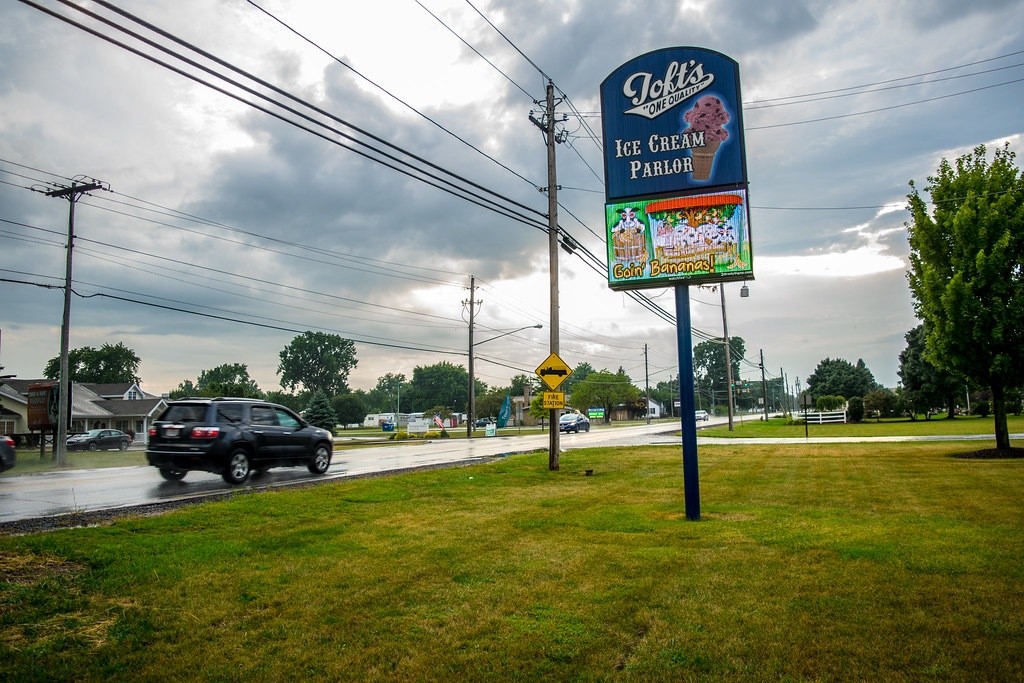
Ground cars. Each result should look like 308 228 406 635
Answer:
695 410 709 422
472 417 493 427
558 413 591 434
0 434 18 475
64 429 133 452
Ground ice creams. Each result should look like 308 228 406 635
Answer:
683 95 729 180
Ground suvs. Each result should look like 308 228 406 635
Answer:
144 397 337 486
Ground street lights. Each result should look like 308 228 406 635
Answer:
465 325 544 437
